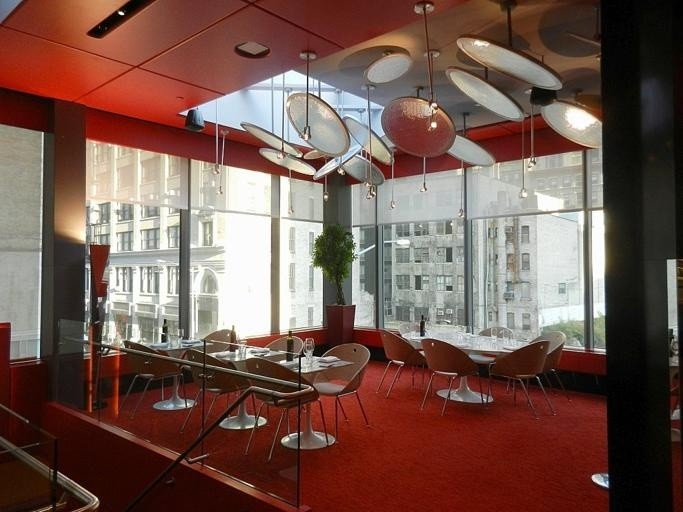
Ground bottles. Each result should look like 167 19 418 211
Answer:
418 314 426 336
285 331 294 361
229 325 237 352
162 319 168 342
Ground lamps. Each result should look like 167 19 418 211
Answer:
414 1 552 199
213 50 413 211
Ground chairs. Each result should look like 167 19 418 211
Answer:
506 332 567 402
312 342 371 445
376 329 433 401
485 340 558 420
420 338 485 416
120 321 303 438
245 358 328 463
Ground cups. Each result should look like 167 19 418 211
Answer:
454 326 515 349
239 339 247 356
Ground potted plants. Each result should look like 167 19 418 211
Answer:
309 222 360 347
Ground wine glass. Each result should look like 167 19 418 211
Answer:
669 339 679 365
303 338 316 366
174 329 184 349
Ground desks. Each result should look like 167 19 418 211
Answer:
276 356 354 449
401 332 531 404
